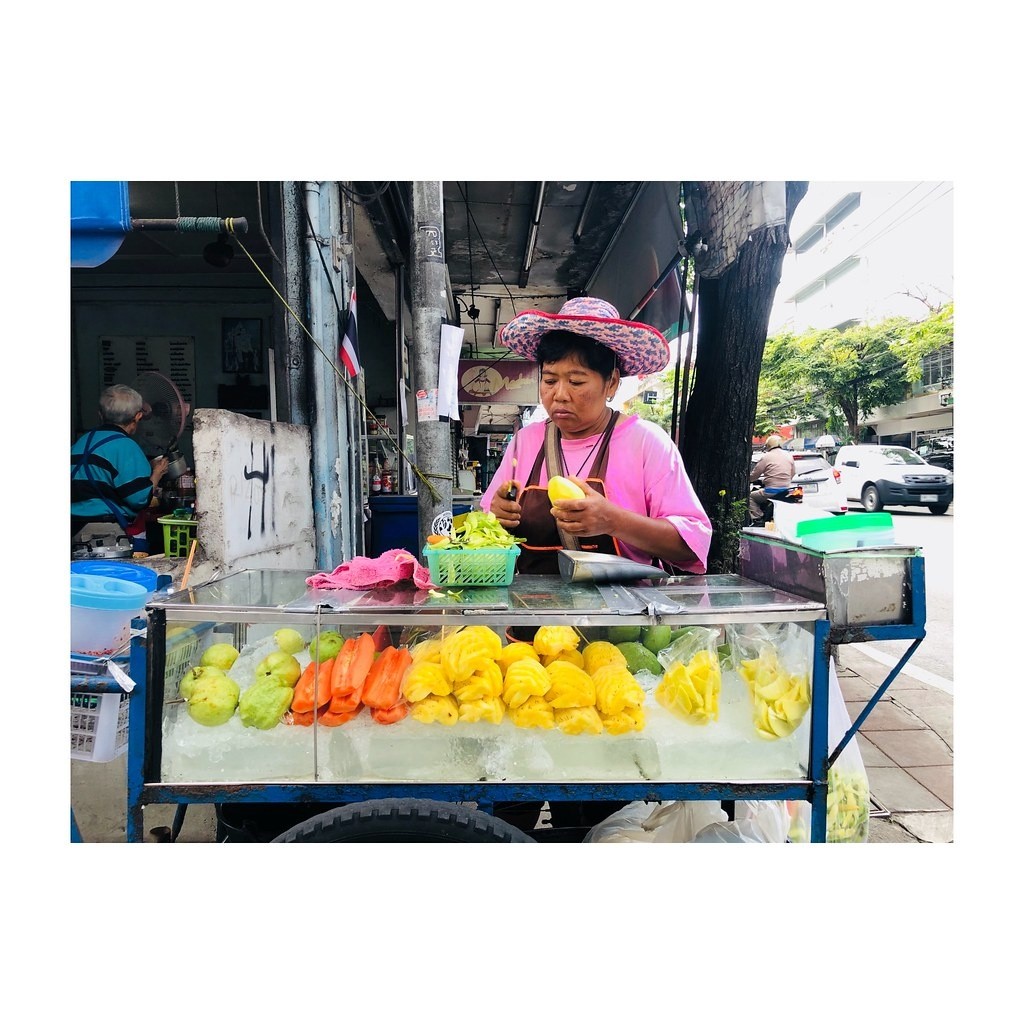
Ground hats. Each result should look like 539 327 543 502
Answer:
497 297 670 375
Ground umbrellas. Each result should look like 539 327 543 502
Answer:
782 438 811 449
804 434 840 459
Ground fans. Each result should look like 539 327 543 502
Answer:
132 371 191 491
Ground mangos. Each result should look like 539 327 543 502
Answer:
608 626 733 677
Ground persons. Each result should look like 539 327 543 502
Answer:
479 297 713 829
71 383 169 538
749 435 796 527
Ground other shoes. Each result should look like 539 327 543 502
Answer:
749 519 765 527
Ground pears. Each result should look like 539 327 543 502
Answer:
179 629 343 729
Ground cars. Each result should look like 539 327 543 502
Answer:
832 445 954 515
750 449 847 515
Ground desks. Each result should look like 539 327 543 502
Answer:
370 494 474 559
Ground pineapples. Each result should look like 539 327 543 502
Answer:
402 626 645 737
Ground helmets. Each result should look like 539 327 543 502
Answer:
765 435 783 451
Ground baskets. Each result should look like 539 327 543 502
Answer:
157 514 199 557
71 622 216 763
422 543 522 586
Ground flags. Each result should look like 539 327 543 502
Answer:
339 292 361 377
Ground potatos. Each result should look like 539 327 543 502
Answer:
547 477 585 509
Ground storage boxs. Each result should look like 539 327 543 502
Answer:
158 514 199 558
70 628 214 763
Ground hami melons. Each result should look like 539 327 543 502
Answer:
290 632 412 727
